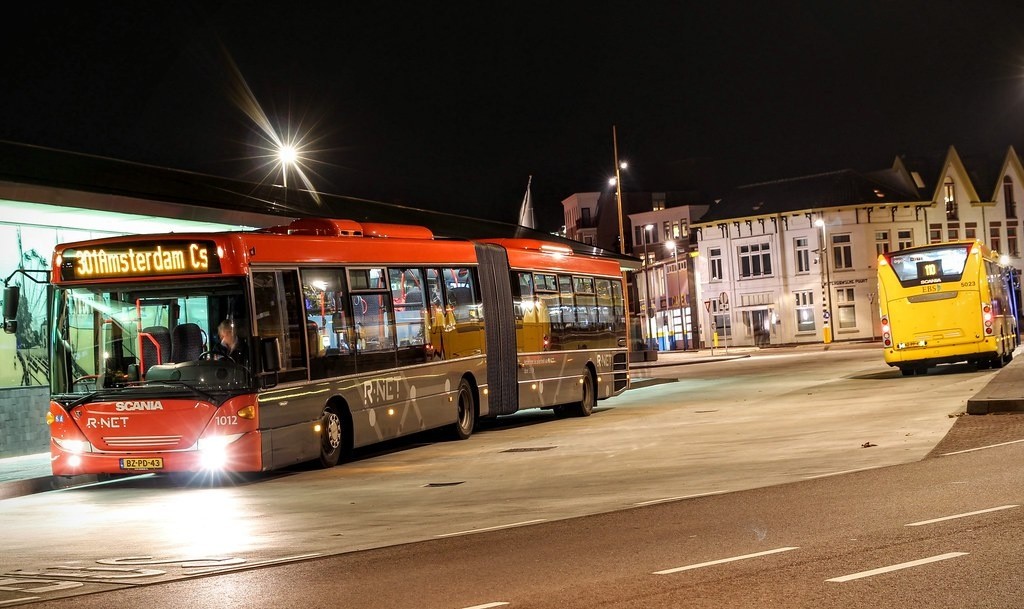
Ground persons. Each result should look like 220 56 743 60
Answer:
208 319 245 361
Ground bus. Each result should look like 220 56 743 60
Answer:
3 217 629 477
877 238 1016 376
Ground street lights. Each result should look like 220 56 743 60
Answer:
814 219 835 342
277 144 299 220
641 223 655 350
667 241 688 350
609 162 628 360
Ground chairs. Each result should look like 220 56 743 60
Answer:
142 326 172 375
172 323 204 364
